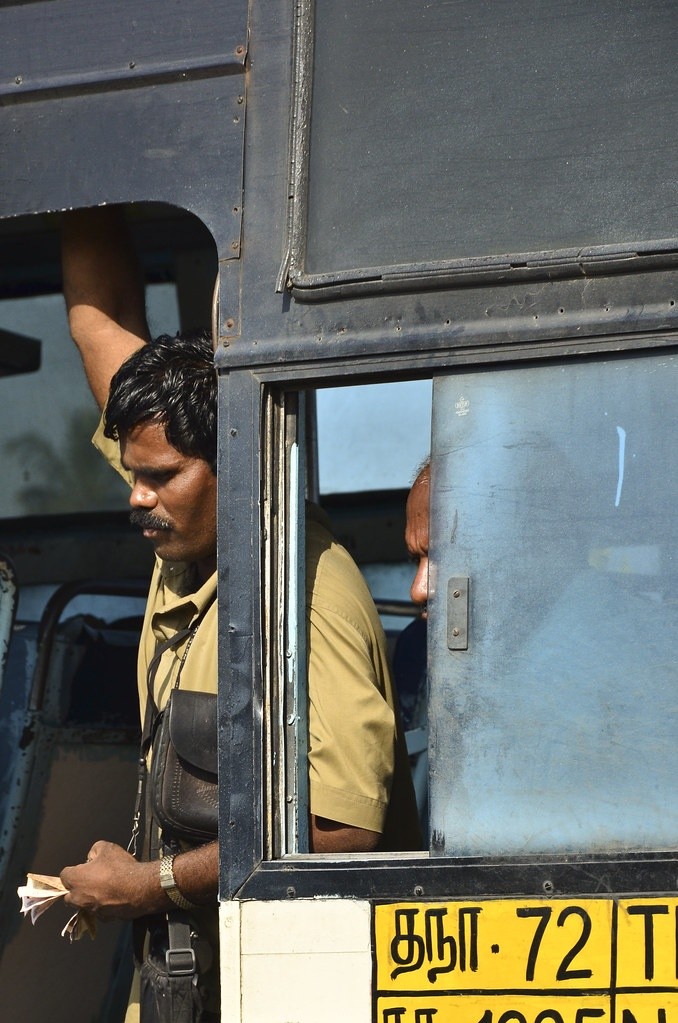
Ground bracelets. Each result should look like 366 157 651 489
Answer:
159 853 203 914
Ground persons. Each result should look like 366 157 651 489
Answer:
405 431 678 854
60 244 423 1023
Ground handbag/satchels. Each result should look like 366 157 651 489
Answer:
148 689 218 840
138 951 206 1023
